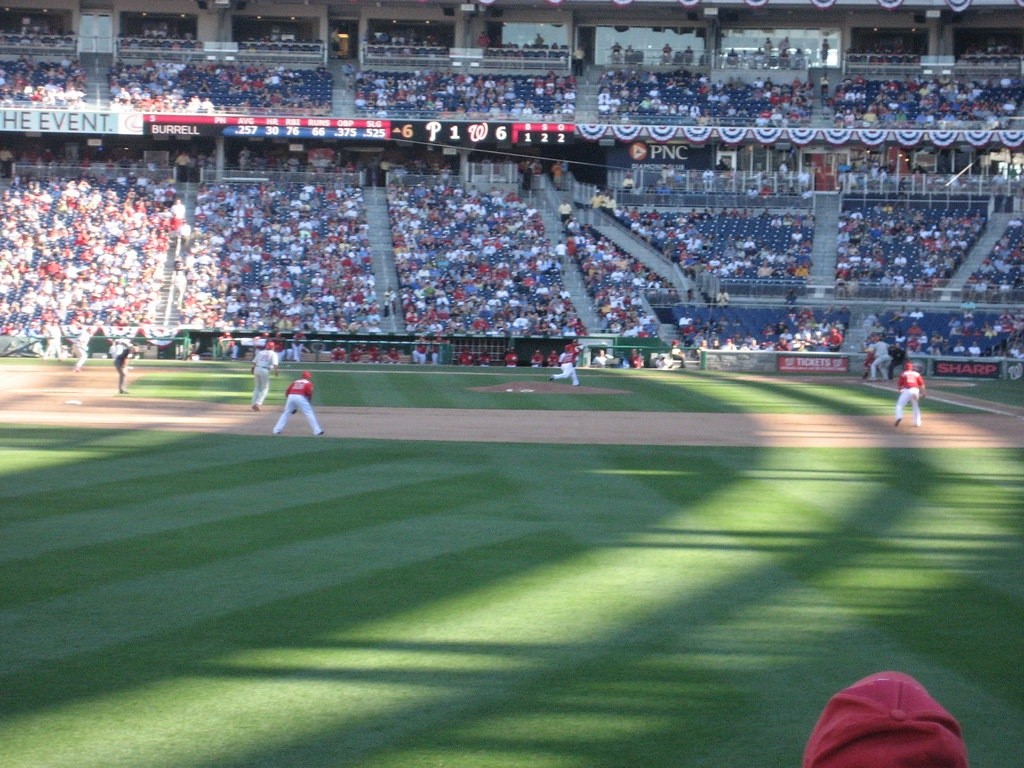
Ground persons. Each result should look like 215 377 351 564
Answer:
894 363 926 426
114 347 133 394
0 34 1024 368
273 371 324 435
804 672 966 768
250 342 278 410
550 345 583 386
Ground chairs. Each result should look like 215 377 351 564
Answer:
843 206 1024 303
836 79 1024 129
671 306 851 351
0 30 578 334
618 211 814 281
597 70 814 122
867 312 1010 356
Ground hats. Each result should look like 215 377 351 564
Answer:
903 361 914 371
301 371 312 380
265 342 275 350
803 671 966 768
564 343 574 353
